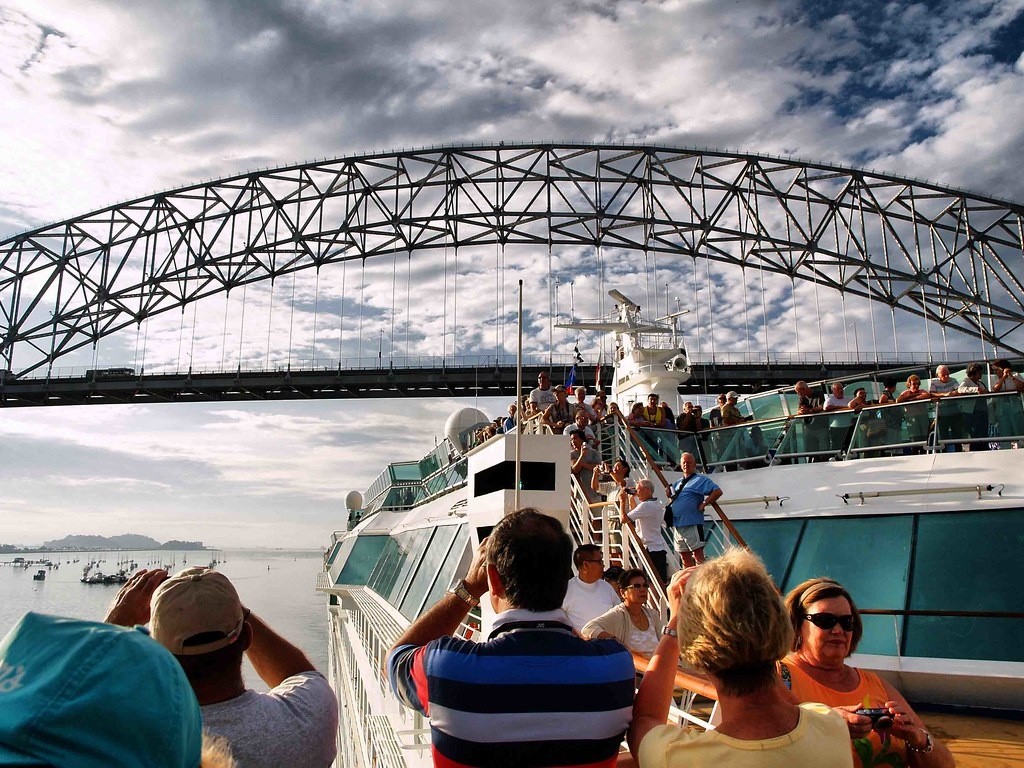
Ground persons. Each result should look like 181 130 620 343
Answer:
0 360 1024 768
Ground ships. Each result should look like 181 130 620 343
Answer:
315 280 1024 767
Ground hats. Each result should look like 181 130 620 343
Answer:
603 566 626 581
149 568 244 655
493 417 502 422
726 391 741 399
552 384 568 397
0 611 202 768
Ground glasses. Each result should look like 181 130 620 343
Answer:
578 417 589 420
719 398 726 399
803 613 854 631
538 377 546 379
687 406 693 408
600 396 607 399
625 583 649 589
584 560 603 565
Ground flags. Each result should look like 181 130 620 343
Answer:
595 353 602 393
572 347 584 365
565 365 577 396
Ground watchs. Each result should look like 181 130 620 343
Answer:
702 500 708 506
449 578 480 608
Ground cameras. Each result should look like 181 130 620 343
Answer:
668 483 676 498
854 707 895 729
743 415 754 422
691 409 697 414
561 419 568 426
599 466 605 472
624 488 637 495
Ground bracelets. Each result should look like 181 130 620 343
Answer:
607 469 613 475
660 626 677 637
244 608 253 621
906 727 934 754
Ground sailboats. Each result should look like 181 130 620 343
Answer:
0 551 232 586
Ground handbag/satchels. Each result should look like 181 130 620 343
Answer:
664 506 673 528
867 418 887 438
